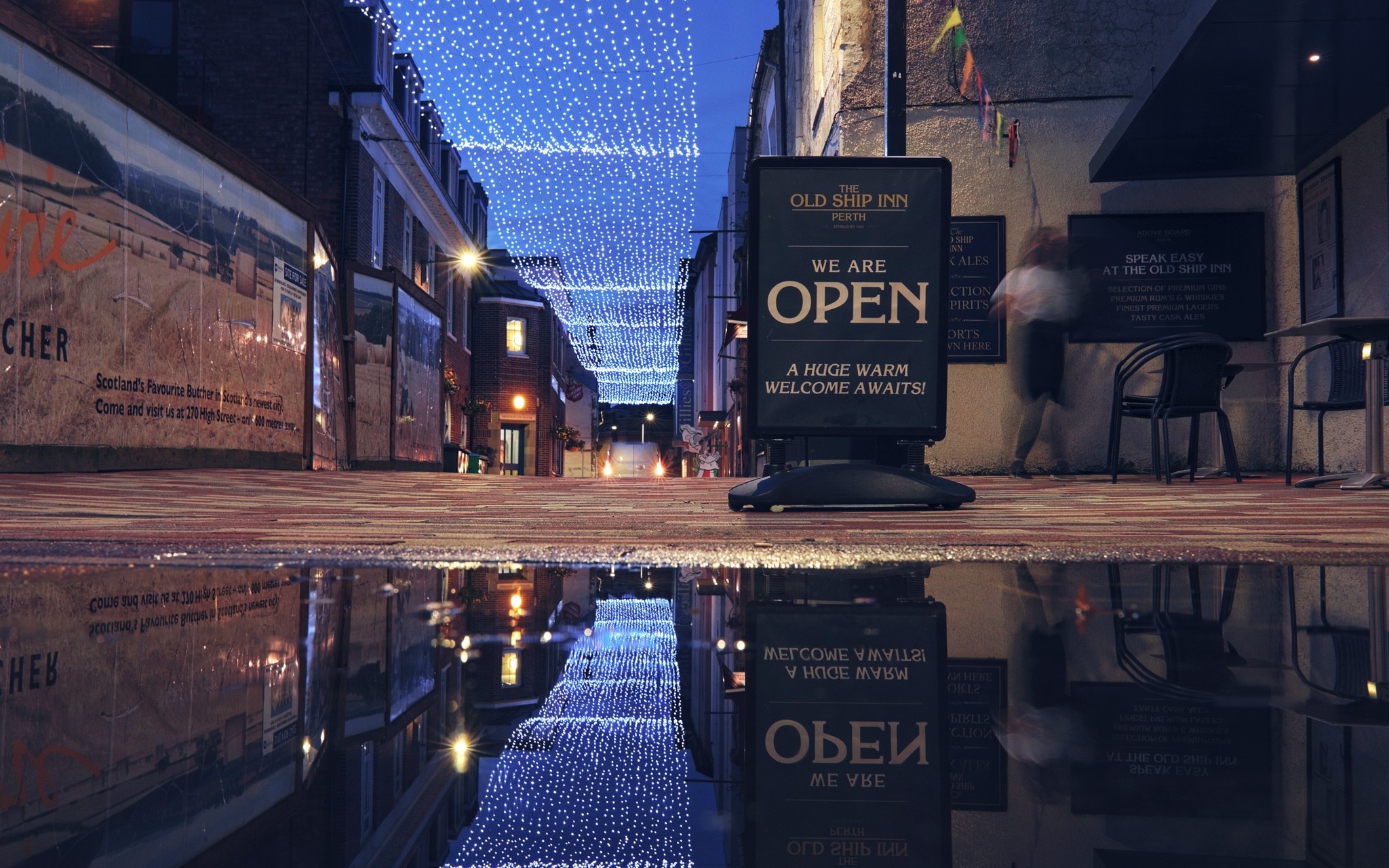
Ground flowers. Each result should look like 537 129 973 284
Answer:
443 369 458 391
472 399 493 412
728 378 741 383
553 568 577 579
558 424 587 446
456 586 487 606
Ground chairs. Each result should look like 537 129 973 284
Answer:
1287 566 1372 704
1109 332 1389 487
1107 561 1242 706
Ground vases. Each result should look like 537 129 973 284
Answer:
731 386 739 391
463 407 474 415
565 447 578 452
555 434 563 440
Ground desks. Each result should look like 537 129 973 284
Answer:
1264 318 1389 489
1269 565 1388 728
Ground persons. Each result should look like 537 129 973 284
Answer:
987 225 1084 475
997 560 1078 793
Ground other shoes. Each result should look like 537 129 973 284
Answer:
1008 461 1035 480
1049 460 1077 480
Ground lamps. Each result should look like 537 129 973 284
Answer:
1362 339 1386 360
